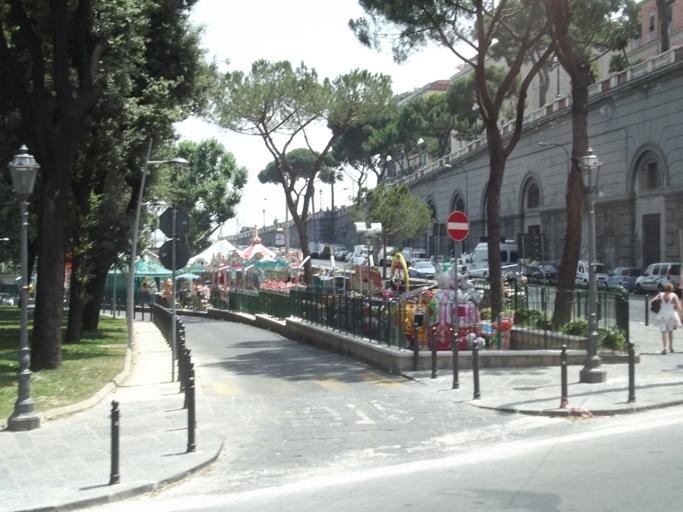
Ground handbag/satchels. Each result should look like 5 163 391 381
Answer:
650 295 662 314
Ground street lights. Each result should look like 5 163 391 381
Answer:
126 135 191 351
5 142 45 433
576 143 607 385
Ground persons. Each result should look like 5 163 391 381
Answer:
647 280 682 355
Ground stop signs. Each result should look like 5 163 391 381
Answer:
446 210 472 243
